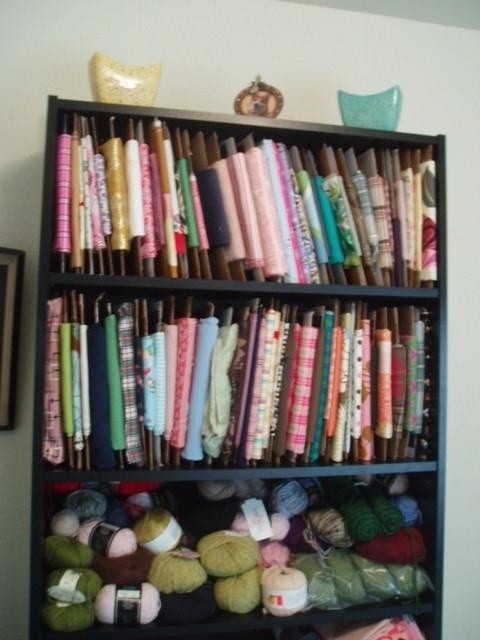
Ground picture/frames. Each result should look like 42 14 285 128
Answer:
3 242 28 435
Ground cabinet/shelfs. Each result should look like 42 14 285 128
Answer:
29 92 451 639
